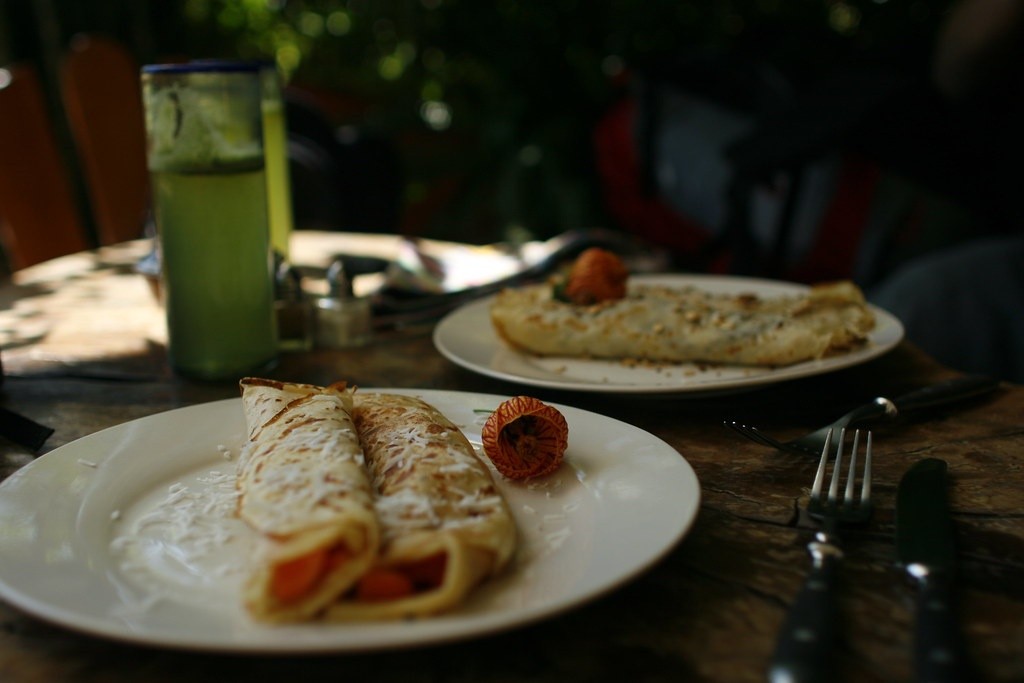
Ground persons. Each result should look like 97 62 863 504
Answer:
867 0 1024 385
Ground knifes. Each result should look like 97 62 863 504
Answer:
891 459 967 683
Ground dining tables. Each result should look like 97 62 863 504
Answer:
0 262 1024 683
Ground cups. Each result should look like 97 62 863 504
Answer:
140 57 292 388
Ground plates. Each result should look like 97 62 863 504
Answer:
433 275 904 391
291 230 524 298
0 389 702 650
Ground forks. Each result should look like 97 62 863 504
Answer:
767 429 872 683
722 373 1001 459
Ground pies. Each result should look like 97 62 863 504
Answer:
490 279 877 366
233 376 518 625
